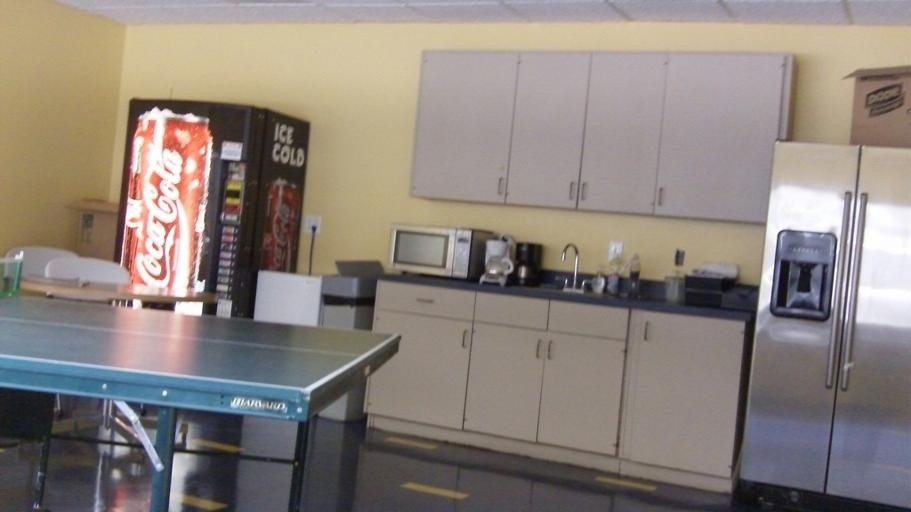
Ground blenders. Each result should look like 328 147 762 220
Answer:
480 238 513 288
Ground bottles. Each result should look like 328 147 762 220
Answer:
607 246 623 296
628 253 642 299
663 248 686 305
119 112 213 299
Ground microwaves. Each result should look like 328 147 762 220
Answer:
388 222 493 282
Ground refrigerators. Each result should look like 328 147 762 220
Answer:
740 141 911 508
114 98 311 319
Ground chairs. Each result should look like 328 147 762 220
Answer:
44 257 146 418
6 246 79 280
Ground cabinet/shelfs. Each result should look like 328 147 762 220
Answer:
653 52 794 223
363 279 476 431
408 50 520 205
505 50 668 215
617 307 745 478
463 292 629 457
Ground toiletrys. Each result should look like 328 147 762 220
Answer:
590 270 606 295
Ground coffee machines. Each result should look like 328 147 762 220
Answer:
515 242 544 286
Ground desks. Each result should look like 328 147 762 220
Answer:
0 292 403 512
18 280 217 303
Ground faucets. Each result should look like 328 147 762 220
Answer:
560 243 579 291
